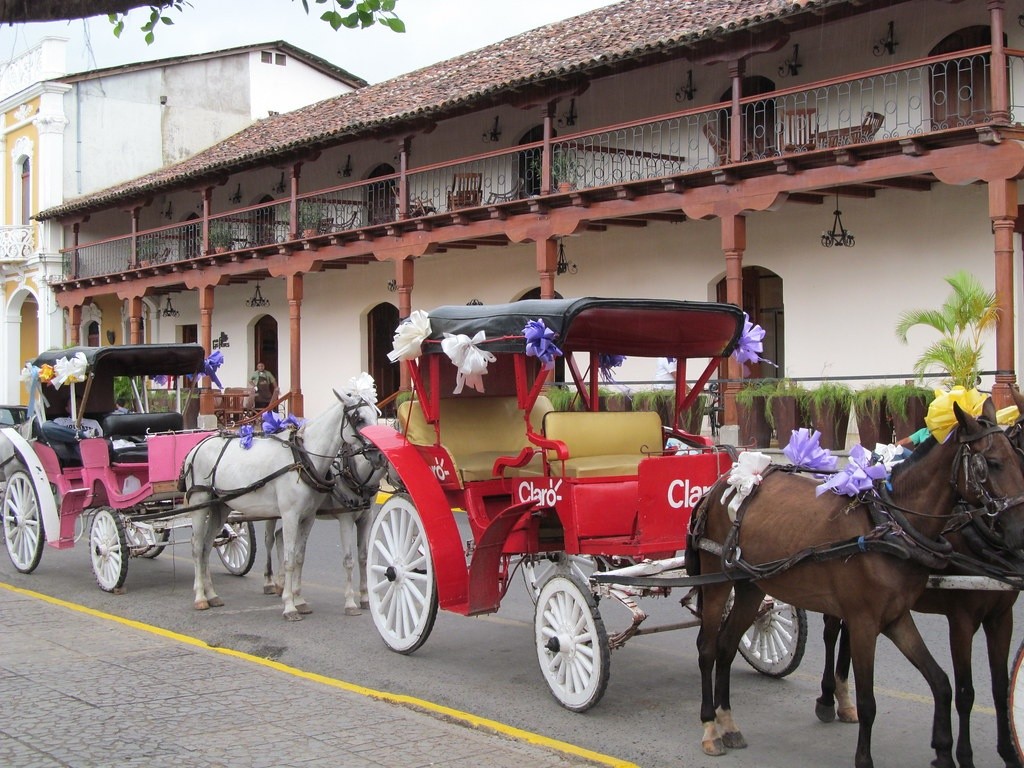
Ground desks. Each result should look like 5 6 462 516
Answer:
784 144 817 153
811 124 871 147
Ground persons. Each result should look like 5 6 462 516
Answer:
115 398 131 414
249 362 277 408
54 394 103 438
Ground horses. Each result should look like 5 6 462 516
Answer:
679 382 1024 768
0 419 38 544
181 386 403 623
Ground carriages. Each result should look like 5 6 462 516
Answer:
363 296 1023 768
1 340 383 620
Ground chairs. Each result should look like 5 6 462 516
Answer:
853 111 885 144
448 172 484 211
784 108 820 145
391 186 437 216
325 211 357 234
485 177 525 208
702 123 754 167
160 247 172 263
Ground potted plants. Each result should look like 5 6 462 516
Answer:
665 388 707 435
135 238 167 268
763 376 811 450
797 382 852 450
208 219 237 254
298 201 327 238
887 384 937 442
545 385 574 412
531 157 580 192
630 390 668 426
735 378 770 448
603 391 631 412
572 387 610 412
852 383 893 452
62 252 84 281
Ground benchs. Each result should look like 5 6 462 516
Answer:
397 392 556 483
543 410 664 478
102 411 184 464
53 413 108 468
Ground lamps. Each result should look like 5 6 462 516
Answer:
482 116 502 144
106 331 115 345
272 171 289 195
778 44 804 78
387 279 397 292
393 153 411 165
196 203 203 211
556 237 569 276
245 280 270 308
820 192 856 248
557 98 578 129
228 183 243 204
160 201 175 220
872 20 899 58
675 69 698 103
161 298 181 318
336 154 353 179
